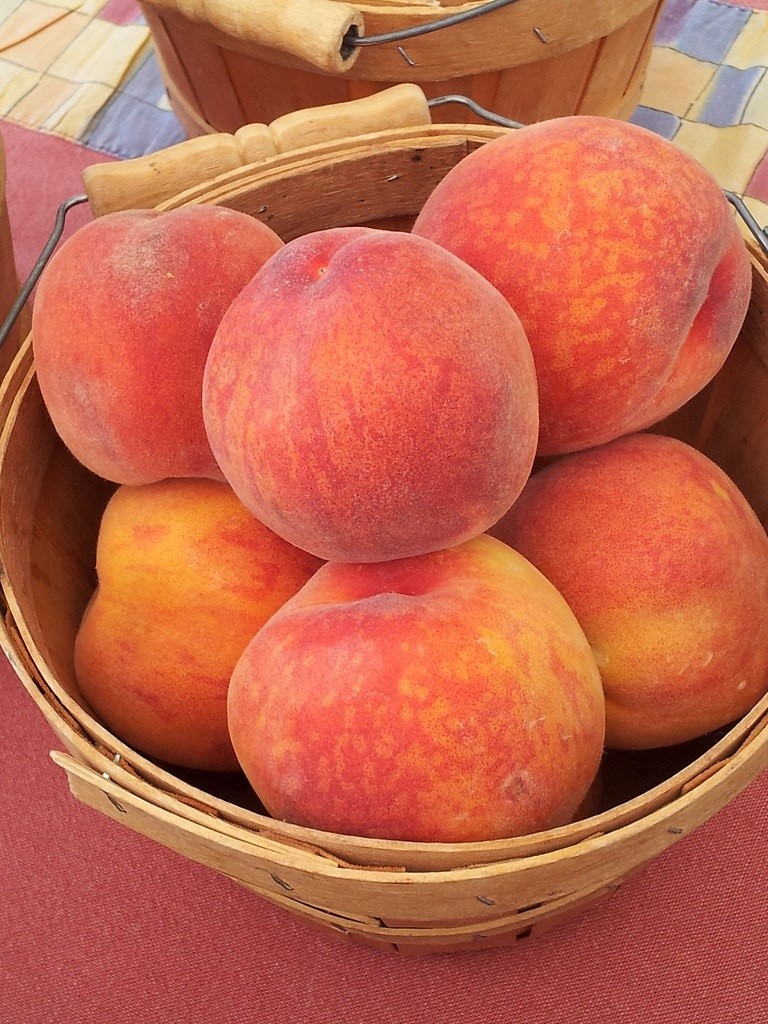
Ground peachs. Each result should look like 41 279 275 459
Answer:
407 115 752 459
226 532 604 844
29 203 288 489
75 479 322 772
201 226 541 567
491 435 768 750
